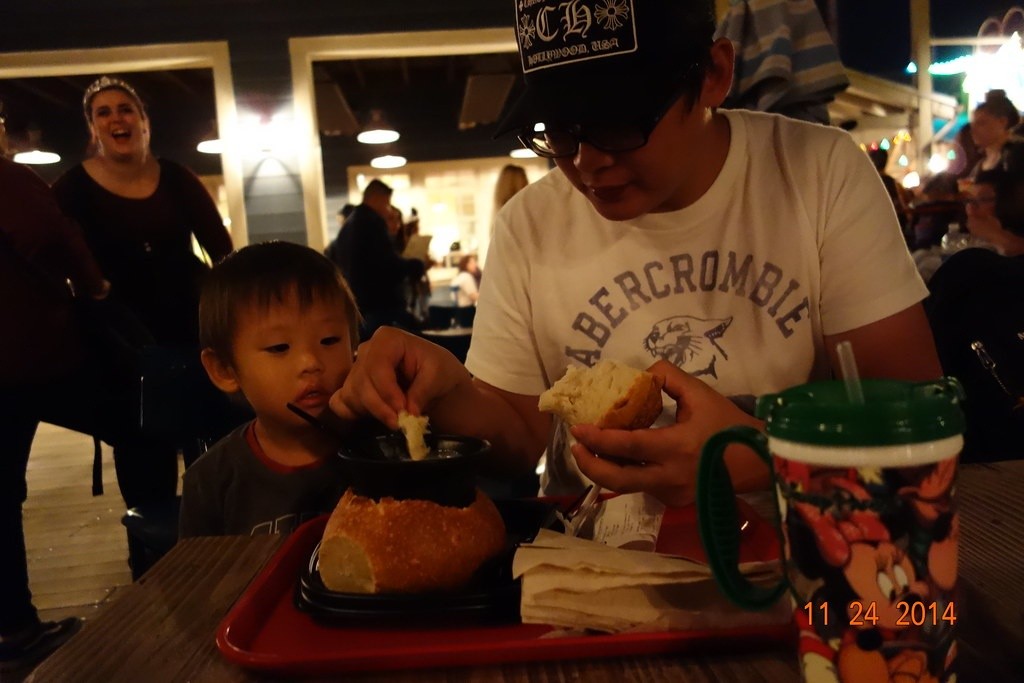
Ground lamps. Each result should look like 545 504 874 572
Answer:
356 112 399 144
196 119 219 153
12 131 61 165
369 144 409 169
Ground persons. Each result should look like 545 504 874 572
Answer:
328 179 432 340
177 242 365 540
0 111 110 672
870 91 1024 259
54 78 234 512
326 0 944 510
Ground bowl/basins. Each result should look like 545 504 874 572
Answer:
334 432 491 506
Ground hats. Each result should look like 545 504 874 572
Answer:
489 0 717 143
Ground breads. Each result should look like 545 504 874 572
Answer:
539 360 664 430
396 409 432 460
317 486 506 595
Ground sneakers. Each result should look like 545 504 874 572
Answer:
0 616 82 671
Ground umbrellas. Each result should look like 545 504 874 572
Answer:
712 0 848 124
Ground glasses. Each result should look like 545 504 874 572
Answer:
517 59 704 158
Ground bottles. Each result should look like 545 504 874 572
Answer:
940 223 967 264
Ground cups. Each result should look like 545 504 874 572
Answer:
754 378 970 683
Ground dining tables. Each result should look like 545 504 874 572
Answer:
29 463 1024 682
